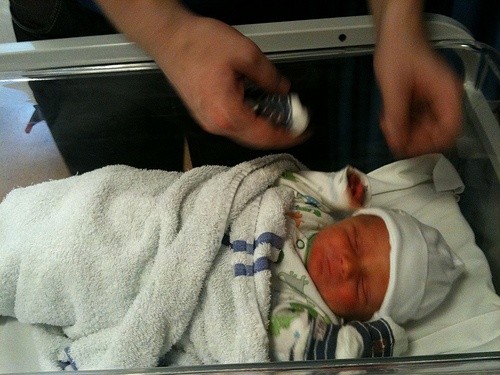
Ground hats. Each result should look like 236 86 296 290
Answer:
351 207 466 325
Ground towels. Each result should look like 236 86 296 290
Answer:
0 154 308 375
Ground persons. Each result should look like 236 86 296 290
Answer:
0 152 466 375
10 0 466 177
